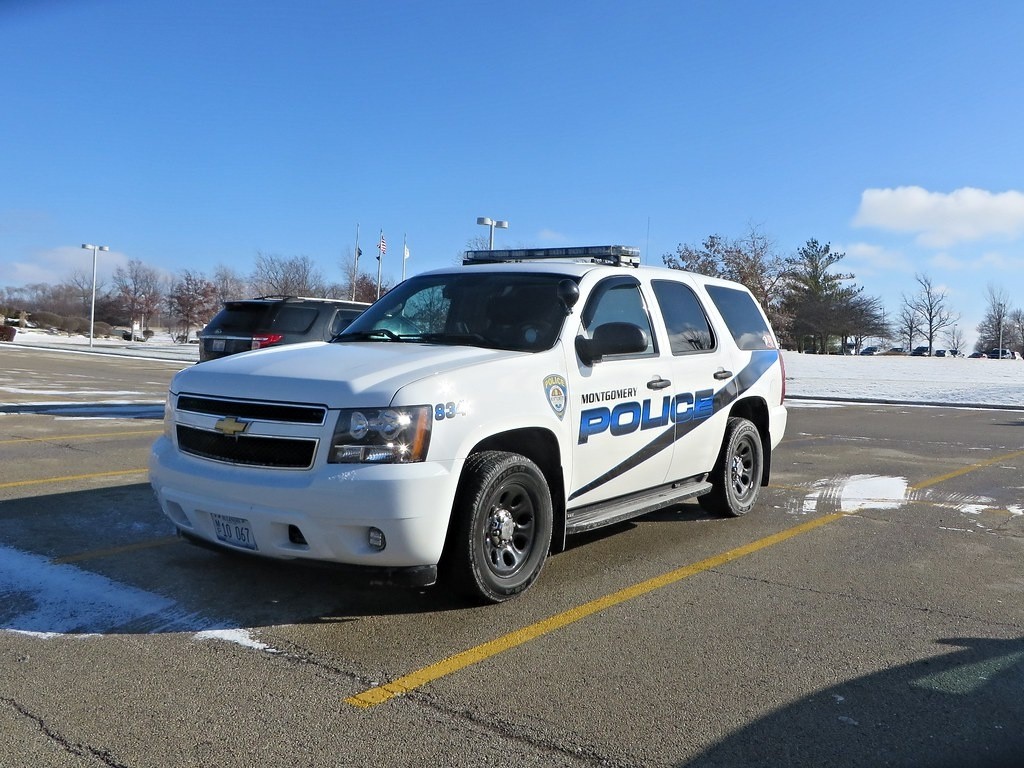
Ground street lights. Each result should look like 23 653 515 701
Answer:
879 307 885 345
476 217 509 251
953 323 958 348
81 243 110 348
997 303 1004 358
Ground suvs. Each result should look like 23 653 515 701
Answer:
193 294 426 367
147 244 786 603
987 348 1012 359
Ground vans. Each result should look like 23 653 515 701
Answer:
842 342 863 355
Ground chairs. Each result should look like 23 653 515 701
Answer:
481 295 550 348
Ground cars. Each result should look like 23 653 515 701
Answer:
889 348 909 353
934 349 954 357
860 346 884 356
968 353 987 358
949 349 965 358
911 346 934 356
1011 351 1023 359
